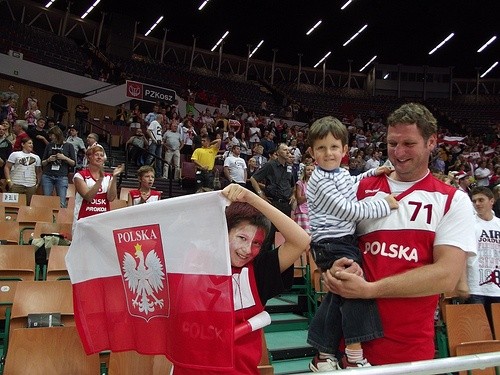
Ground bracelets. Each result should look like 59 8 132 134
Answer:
257 190 263 195
6 178 11 183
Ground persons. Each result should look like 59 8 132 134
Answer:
72 146 124 235
294 162 315 264
162 122 184 183
127 165 162 206
250 142 297 247
41 125 76 208
168 183 311 375
305 116 399 373
4 137 42 206
144 114 164 181
320 103 478 375
455 186 500 340
116 71 319 171
318 108 500 219
75 99 89 137
191 134 221 193
223 145 247 188
83 133 107 171
0 48 111 193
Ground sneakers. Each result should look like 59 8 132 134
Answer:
309 351 371 373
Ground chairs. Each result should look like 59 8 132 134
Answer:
0 18 500 374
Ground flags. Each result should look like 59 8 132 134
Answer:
64 189 234 370
